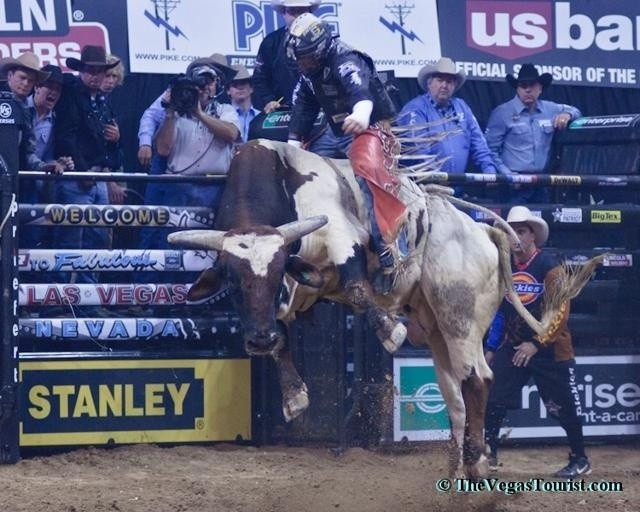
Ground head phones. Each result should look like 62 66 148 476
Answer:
188 63 226 91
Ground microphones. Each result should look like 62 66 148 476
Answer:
208 86 225 99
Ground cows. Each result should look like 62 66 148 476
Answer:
167 138 611 486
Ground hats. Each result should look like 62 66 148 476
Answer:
191 66 219 85
41 64 71 89
506 64 553 90
0 52 53 83
271 0 322 12
506 204 549 245
231 63 252 82
417 57 467 93
186 52 238 84
66 45 121 73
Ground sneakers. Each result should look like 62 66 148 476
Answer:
372 264 399 297
481 457 499 471
552 452 592 478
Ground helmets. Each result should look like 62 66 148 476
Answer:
286 13 337 76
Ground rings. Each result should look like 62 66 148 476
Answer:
561 119 565 121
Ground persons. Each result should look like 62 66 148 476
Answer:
285 12 412 295
0 44 126 317
134 53 263 358
484 206 592 478
250 0 322 113
485 64 582 204
398 58 497 197
